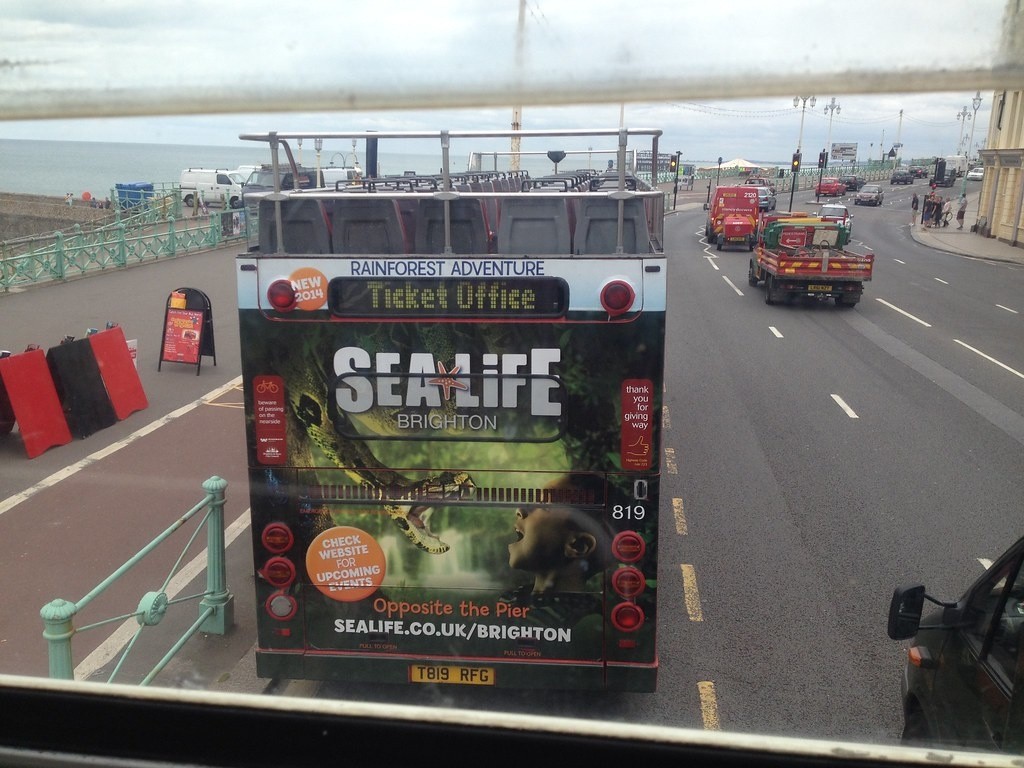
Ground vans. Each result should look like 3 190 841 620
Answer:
242 165 326 217
180 167 249 208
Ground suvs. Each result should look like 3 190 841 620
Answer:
746 177 775 196
813 203 855 245
928 167 957 188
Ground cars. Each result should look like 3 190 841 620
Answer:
909 165 929 178
883 536 1023 758
838 175 866 191
755 186 777 212
855 184 884 206
890 169 915 185
966 167 984 181
814 178 848 197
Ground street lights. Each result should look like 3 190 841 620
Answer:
956 105 972 155
823 97 841 152
791 94 817 192
956 90 982 204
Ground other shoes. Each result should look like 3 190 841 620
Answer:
924 223 949 228
957 226 963 229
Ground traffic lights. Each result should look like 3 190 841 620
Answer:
791 152 802 173
818 151 828 168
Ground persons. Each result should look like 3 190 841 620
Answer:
507 471 647 662
909 193 919 227
607 160 614 171
921 191 951 228
64 193 112 209
956 193 968 230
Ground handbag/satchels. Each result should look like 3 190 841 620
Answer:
942 214 946 221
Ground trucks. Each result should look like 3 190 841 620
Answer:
747 209 875 308
702 185 760 250
